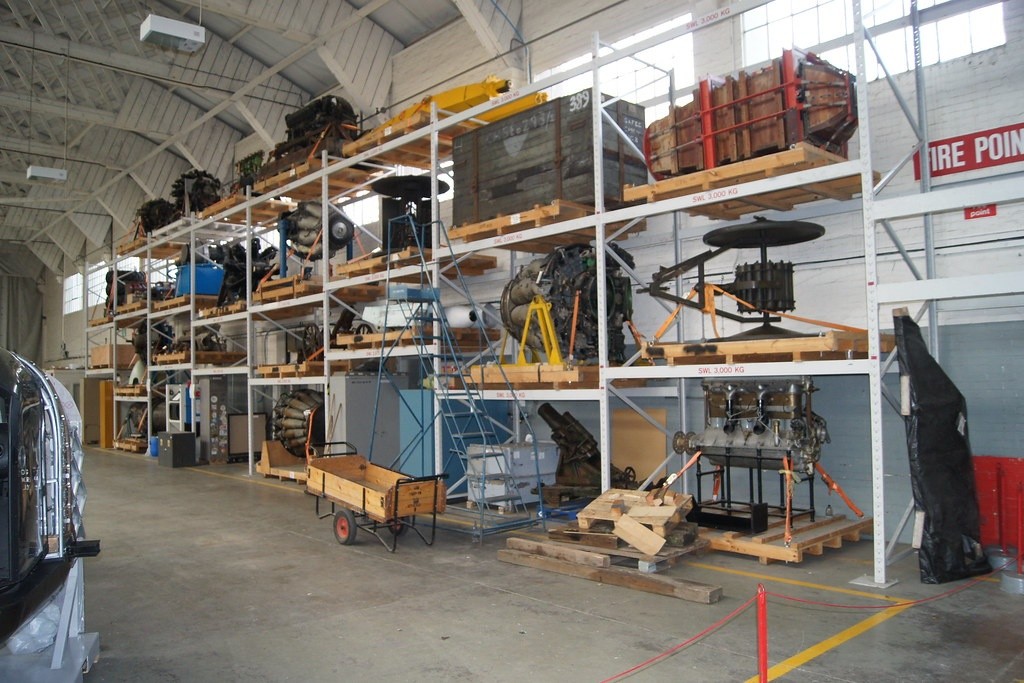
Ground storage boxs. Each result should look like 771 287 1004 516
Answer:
302 455 446 523
452 86 648 233
173 262 225 297
90 343 139 369
156 430 195 469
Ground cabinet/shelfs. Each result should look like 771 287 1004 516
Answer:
84 0 943 593
466 443 561 506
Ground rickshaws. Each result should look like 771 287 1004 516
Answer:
304 441 449 553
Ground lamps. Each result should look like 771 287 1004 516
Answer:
25 31 72 186
140 0 206 54
